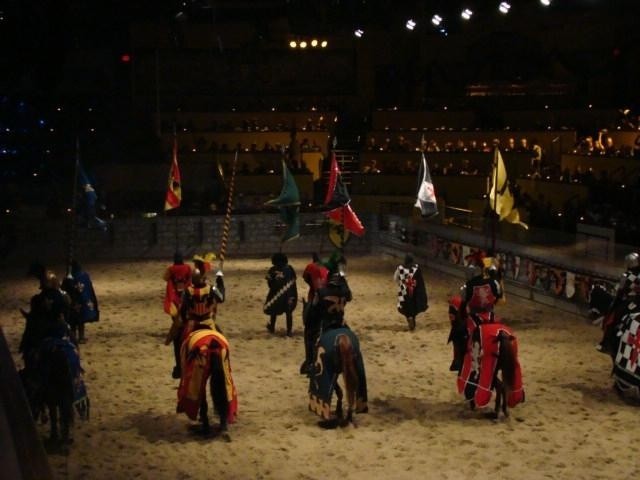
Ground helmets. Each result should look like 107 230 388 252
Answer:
462 254 484 280
481 256 501 272
40 271 60 292
328 250 348 281
404 249 416 267
624 250 640 271
173 249 184 265
192 267 210 286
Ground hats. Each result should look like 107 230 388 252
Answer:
270 250 288 269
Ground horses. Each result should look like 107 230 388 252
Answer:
301 296 365 428
446 292 518 422
588 283 640 399
17 305 75 457
170 313 233 443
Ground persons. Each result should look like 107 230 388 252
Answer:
61 260 99 345
20 269 81 378
446 257 504 372
595 252 640 353
302 251 329 320
3 118 639 213
263 252 297 338
171 255 226 378
393 255 429 332
164 252 194 324
300 250 353 375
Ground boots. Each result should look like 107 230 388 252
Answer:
266 310 277 333
406 314 416 333
171 336 182 378
596 322 612 352
285 311 293 337
449 330 465 371
46 404 59 444
76 323 88 344
60 405 75 444
301 324 316 371
